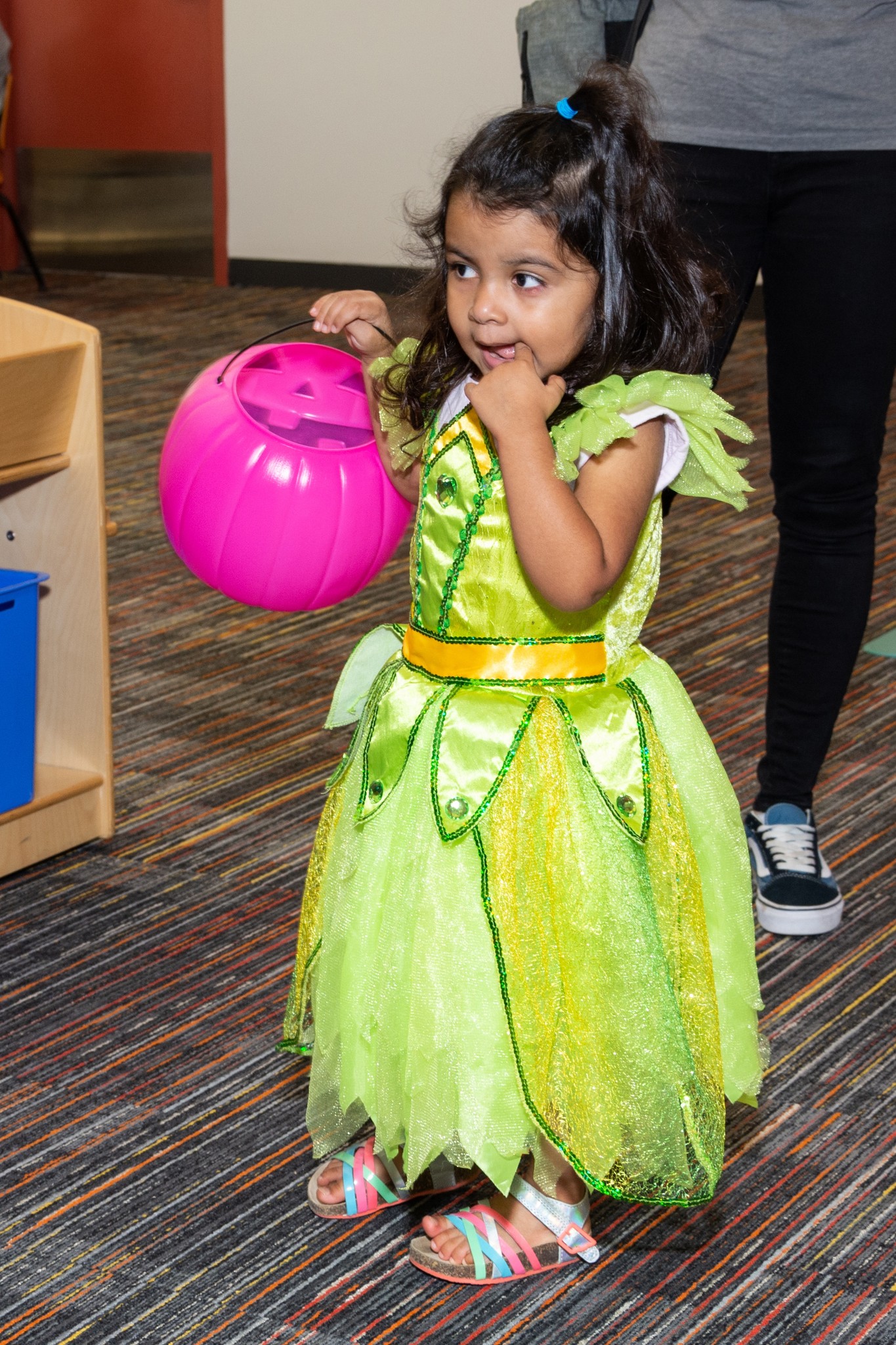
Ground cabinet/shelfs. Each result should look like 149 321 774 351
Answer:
0 293 115 884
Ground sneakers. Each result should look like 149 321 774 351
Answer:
741 799 844 936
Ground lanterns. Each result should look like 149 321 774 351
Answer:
157 340 410 611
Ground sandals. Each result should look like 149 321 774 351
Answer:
306 1132 482 1218
407 1170 600 1286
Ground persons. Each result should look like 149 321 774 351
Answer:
617 0 896 934
274 63 768 1286
